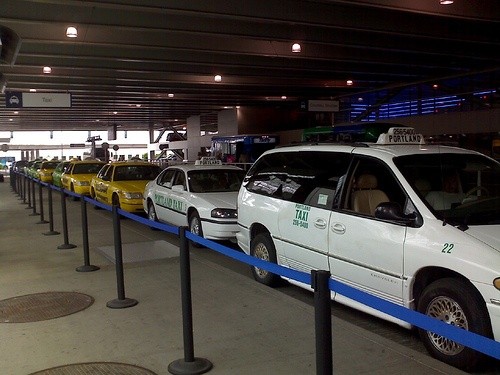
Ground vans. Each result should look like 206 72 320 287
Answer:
235 143 500 369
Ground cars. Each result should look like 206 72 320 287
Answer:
90 161 163 214
12 159 108 200
142 164 245 248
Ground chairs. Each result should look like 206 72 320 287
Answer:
351 175 390 216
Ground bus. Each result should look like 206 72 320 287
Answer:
300 122 415 146
209 134 280 171
